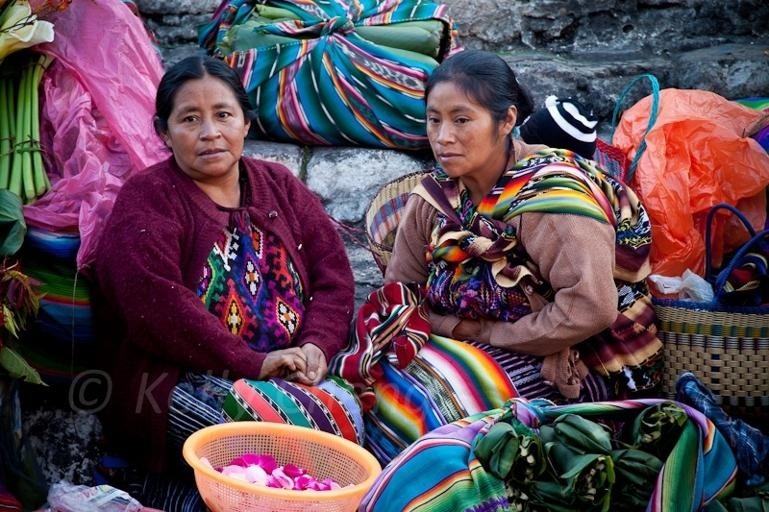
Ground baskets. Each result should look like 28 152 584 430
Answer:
182 421 383 512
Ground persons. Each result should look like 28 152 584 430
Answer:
96 55 367 447
367 50 625 405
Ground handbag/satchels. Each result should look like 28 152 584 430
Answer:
651 203 769 407
326 169 435 278
591 73 661 185
